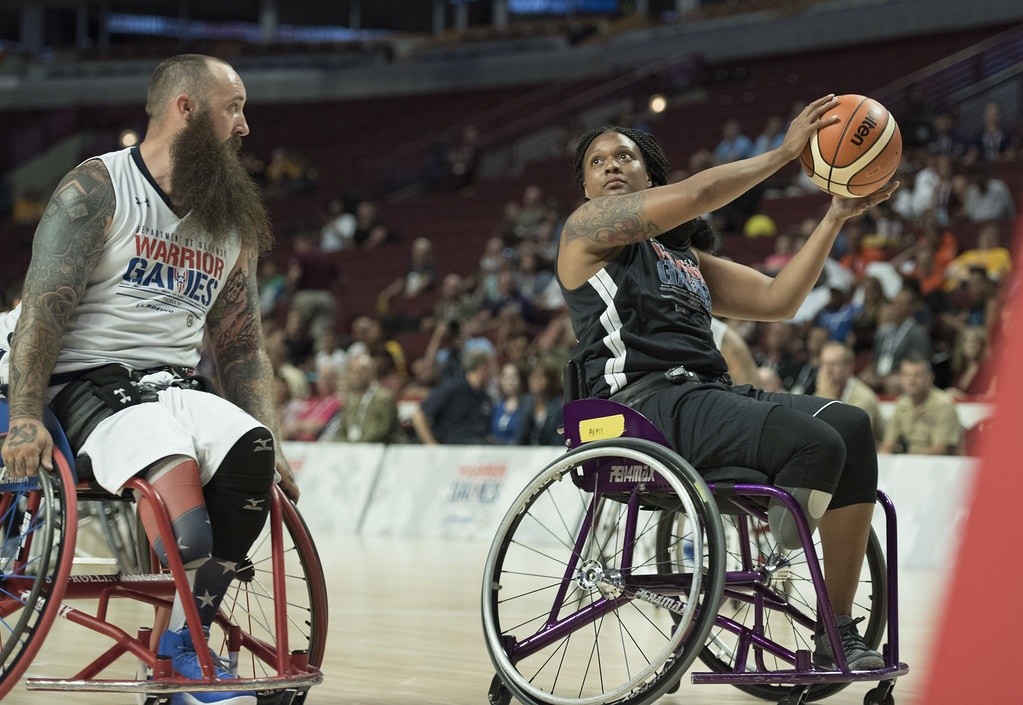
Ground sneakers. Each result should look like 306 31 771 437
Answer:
149 624 257 705
810 616 885 671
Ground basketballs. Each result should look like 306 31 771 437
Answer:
796 92 905 200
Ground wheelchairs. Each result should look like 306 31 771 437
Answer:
0 398 328 705
480 356 909 705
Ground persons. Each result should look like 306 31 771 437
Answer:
377 237 452 311
556 94 885 672
0 55 299 704
879 352 966 455
811 341 884 442
0 97 1023 450
411 337 499 445
